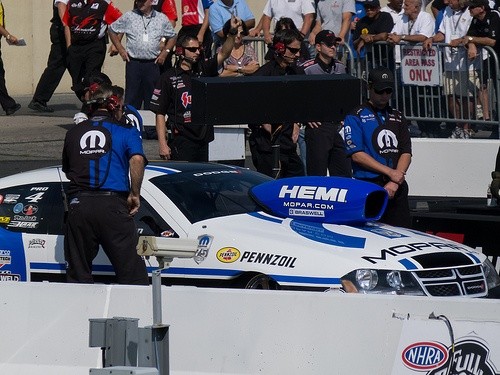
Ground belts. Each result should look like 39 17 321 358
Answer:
70 190 128 197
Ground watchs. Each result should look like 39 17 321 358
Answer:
165 49 170 52
468 37 472 42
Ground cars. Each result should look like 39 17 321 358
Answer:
0 160 500 300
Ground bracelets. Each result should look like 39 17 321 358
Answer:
237 66 241 72
7 34 10 39
401 35 405 39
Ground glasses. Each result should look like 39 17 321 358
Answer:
374 88 393 95
119 102 125 113
182 45 201 53
285 44 302 54
319 42 340 47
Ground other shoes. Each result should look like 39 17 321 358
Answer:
28 99 54 112
6 103 21 115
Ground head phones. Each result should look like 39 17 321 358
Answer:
85 97 120 110
273 30 289 54
175 34 202 55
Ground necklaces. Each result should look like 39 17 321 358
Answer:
230 44 245 65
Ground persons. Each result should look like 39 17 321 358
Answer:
344 66 412 228
244 0 356 177
149 11 241 161
27 0 71 112
62 0 124 100
346 0 500 140
490 146 500 199
109 0 177 110
60 94 148 284
0 2 21 115
70 72 143 131
153 0 260 77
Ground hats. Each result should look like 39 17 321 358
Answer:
315 30 342 42
362 0 380 9
464 0 489 7
368 66 396 91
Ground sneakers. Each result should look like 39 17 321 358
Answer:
447 126 464 138
457 129 477 139
